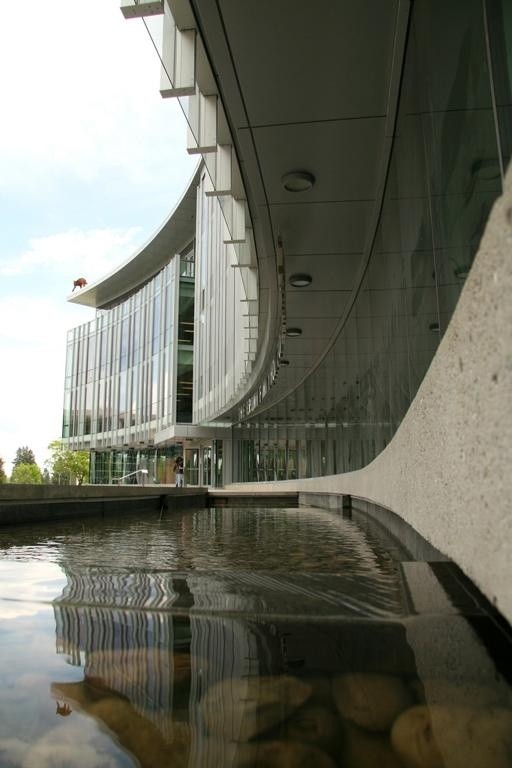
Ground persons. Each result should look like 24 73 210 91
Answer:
173 457 183 487
